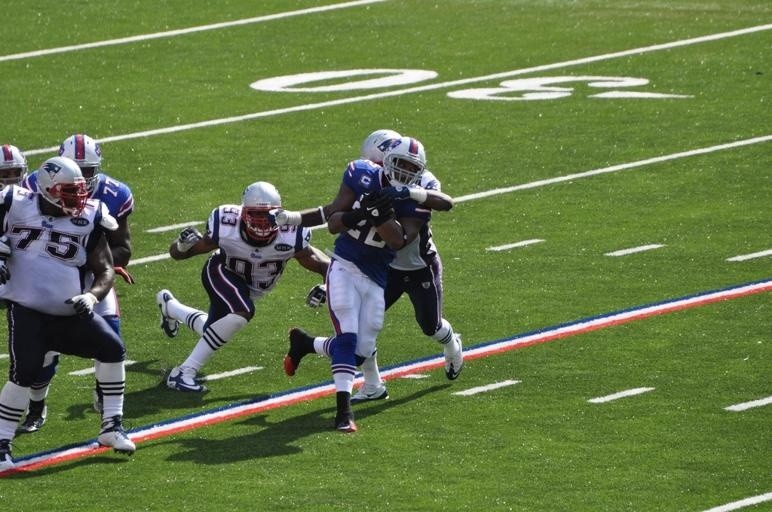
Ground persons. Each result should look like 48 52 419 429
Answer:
158 181 331 392
20 135 134 435
267 136 432 431
0 156 137 473
349 130 462 404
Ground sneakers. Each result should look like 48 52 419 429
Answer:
156 288 181 339
92 385 104 414
96 417 138 457
282 325 311 376
443 332 466 382
349 384 389 404
334 410 359 434
16 405 49 434
165 366 210 394
0 438 16 472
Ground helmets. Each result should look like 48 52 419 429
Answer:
58 132 105 176
379 133 429 172
241 179 285 216
359 128 408 170
35 155 89 215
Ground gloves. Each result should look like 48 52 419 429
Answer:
0 262 12 286
62 290 101 321
305 282 327 309
175 226 204 254
366 183 412 213
359 191 397 229
265 206 293 231
